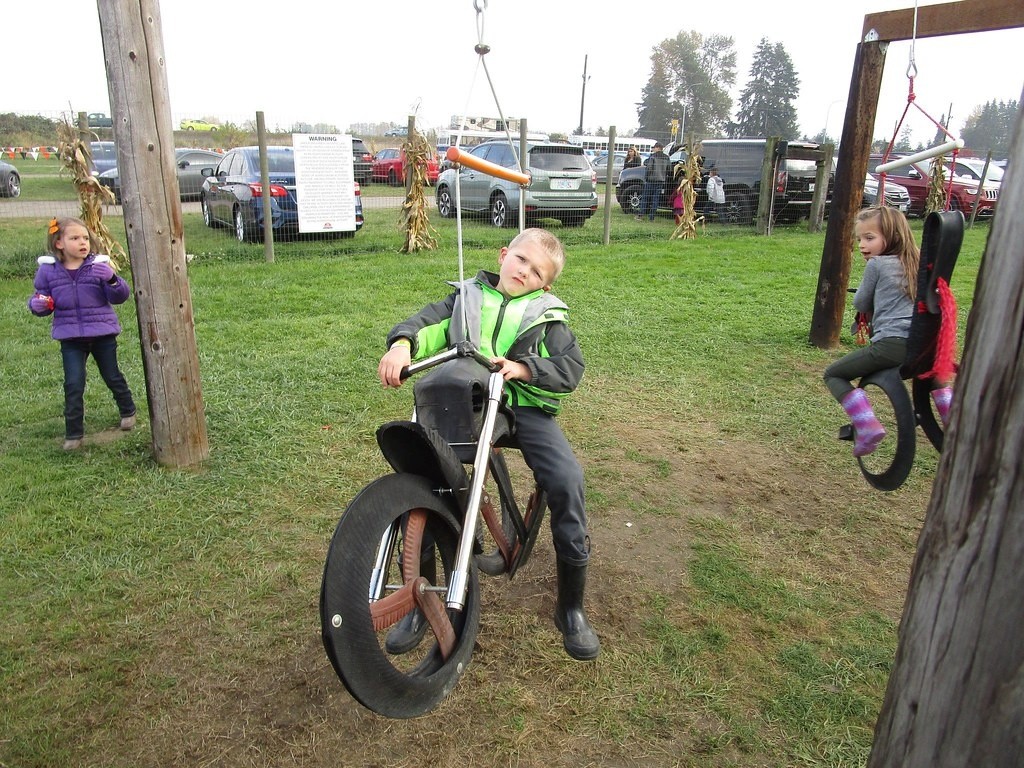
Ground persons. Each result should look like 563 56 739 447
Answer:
29 216 131 449
623 146 641 169
823 205 957 457
699 166 726 224
666 184 684 224
634 142 671 222
378 227 601 661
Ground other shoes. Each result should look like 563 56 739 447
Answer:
634 215 643 222
648 219 654 222
120 412 137 430
63 437 84 451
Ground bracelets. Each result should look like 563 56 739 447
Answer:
390 341 410 349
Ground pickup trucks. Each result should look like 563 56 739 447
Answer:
73 113 112 128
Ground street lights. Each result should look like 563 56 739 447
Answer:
678 82 703 144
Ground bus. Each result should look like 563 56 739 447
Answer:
432 127 550 162
567 135 657 161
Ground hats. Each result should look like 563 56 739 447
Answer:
652 142 663 149
708 167 719 172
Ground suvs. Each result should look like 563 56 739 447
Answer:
867 151 1001 221
615 139 837 227
352 138 374 184
434 139 599 229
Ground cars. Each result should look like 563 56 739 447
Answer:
383 125 408 137
439 145 474 173
0 159 21 198
373 147 440 187
832 157 911 218
941 155 1008 182
96 147 225 204
591 153 649 184
180 119 221 132
199 146 365 244
91 140 117 180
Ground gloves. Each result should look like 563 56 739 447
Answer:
30 298 48 313
91 262 113 282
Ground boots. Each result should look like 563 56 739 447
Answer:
383 542 439 655
840 388 885 458
928 386 960 430
552 548 600 661
